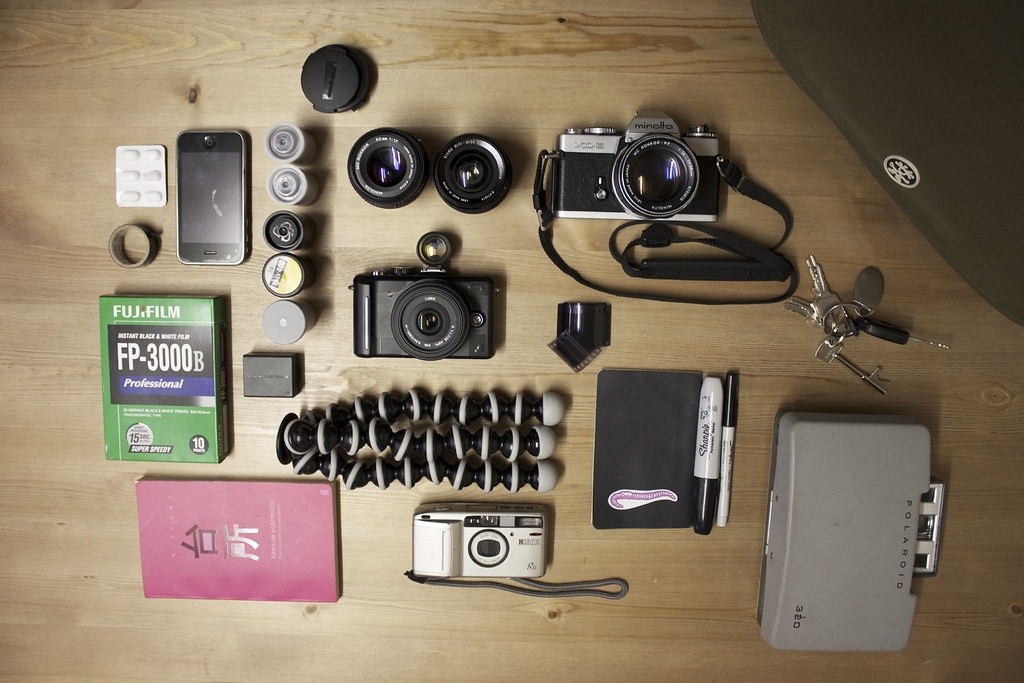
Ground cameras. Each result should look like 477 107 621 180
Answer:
413 501 550 578
348 231 501 361
549 109 721 221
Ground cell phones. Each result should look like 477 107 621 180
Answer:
174 131 247 265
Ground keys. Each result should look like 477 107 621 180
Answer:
785 254 951 396
